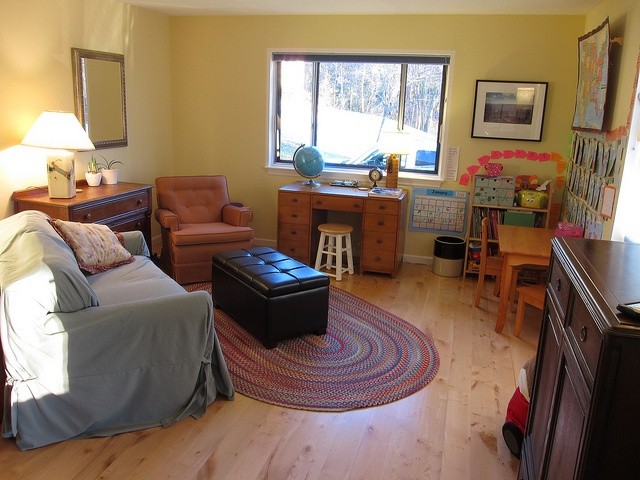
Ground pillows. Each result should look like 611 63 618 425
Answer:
48 218 133 274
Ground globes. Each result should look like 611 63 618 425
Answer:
293 144 323 186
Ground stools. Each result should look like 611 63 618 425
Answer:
314 223 355 281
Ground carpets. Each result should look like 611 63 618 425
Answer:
186 284 439 411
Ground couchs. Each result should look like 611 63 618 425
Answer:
0 210 235 452
155 174 253 286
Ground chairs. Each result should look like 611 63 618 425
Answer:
474 216 523 310
514 284 543 336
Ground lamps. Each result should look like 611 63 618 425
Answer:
20 109 95 198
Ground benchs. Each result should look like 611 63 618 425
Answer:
212 244 330 348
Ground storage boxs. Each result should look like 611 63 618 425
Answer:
475 176 515 206
504 212 535 226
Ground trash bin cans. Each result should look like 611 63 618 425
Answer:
432 235 466 277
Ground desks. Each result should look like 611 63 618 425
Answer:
495 223 555 335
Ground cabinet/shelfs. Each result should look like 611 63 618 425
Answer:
10 180 154 258
278 182 408 280
520 237 640 480
463 181 553 279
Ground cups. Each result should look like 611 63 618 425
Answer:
335 181 343 185
344 182 352 185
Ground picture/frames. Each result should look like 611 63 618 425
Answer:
471 79 549 141
70 47 128 151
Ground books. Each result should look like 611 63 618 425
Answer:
472 207 503 240
369 185 404 200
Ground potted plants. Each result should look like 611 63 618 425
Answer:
95 155 122 186
85 156 103 187
